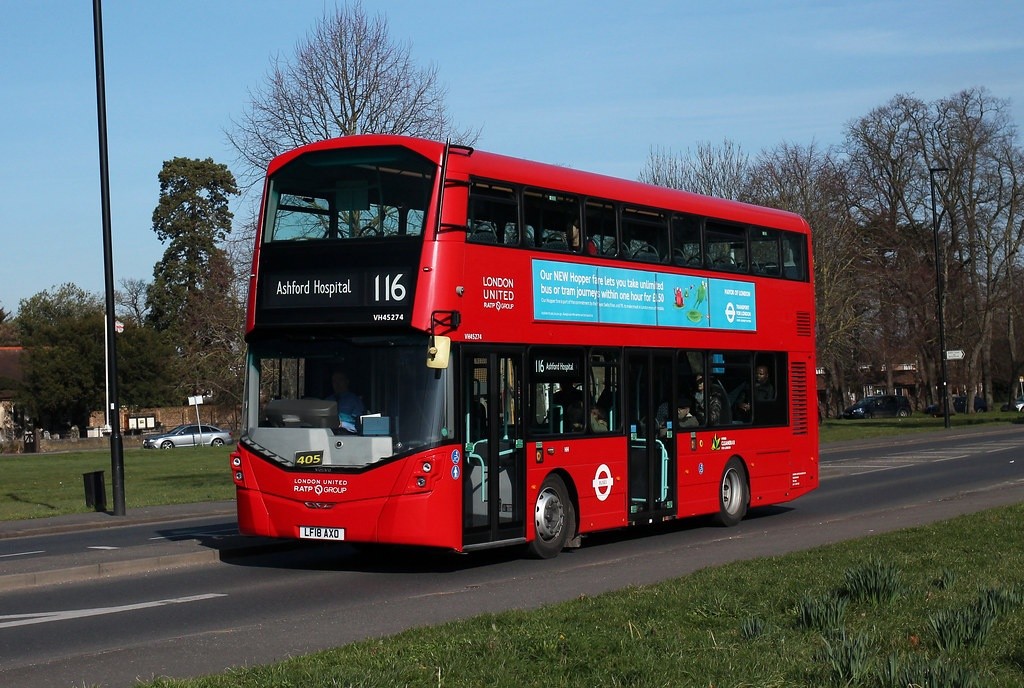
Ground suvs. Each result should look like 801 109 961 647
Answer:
843 394 914 419
926 395 987 419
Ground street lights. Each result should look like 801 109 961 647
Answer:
927 166 947 429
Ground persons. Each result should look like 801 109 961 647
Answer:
546 365 774 434
323 366 364 432
566 217 597 255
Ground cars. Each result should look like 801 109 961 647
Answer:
142 424 234 451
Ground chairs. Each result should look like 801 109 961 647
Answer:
468 223 780 277
324 225 401 237
707 373 773 424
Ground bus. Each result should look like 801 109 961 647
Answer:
230 134 819 565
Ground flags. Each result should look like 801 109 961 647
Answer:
114 319 124 333
